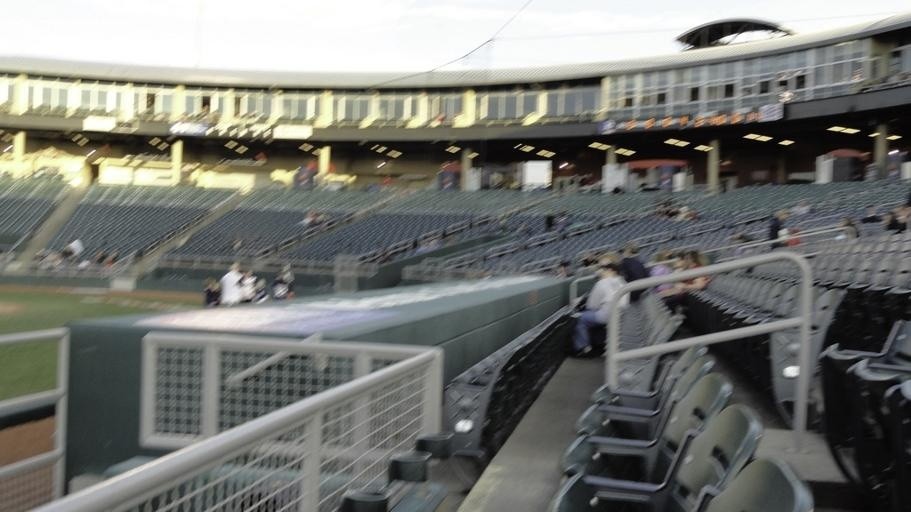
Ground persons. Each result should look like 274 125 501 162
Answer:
2 98 911 362
